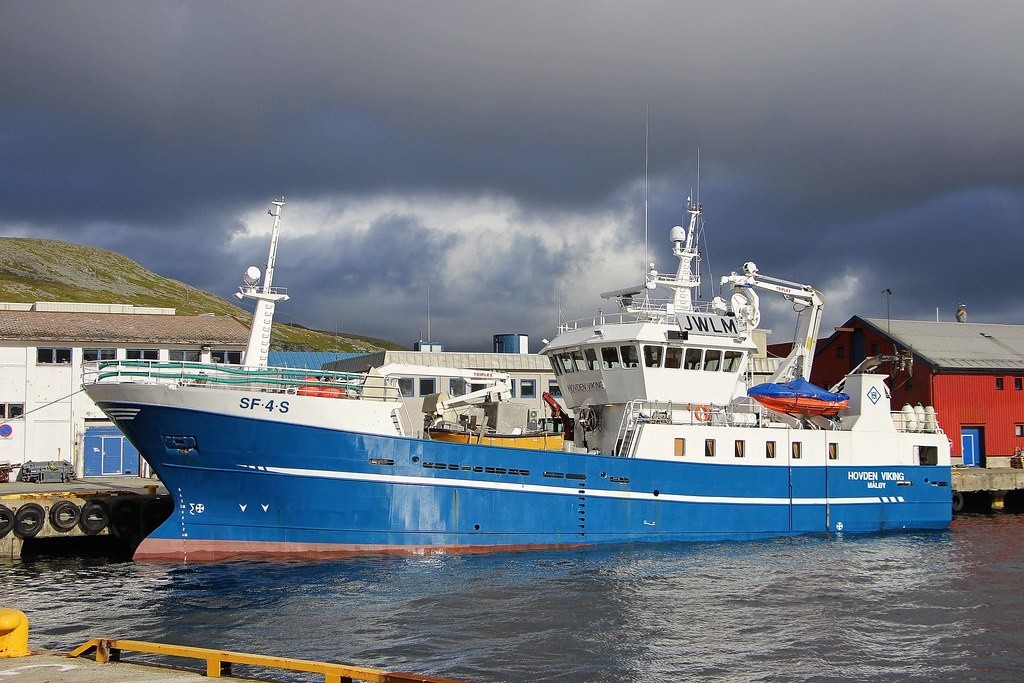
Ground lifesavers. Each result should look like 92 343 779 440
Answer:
695 404 711 421
578 407 598 431
14 502 45 540
0 503 15 540
49 499 82 532
80 499 170 541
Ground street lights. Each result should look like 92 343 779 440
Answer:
880 286 894 333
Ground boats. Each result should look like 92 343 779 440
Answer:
77 147 957 562
745 377 849 417
